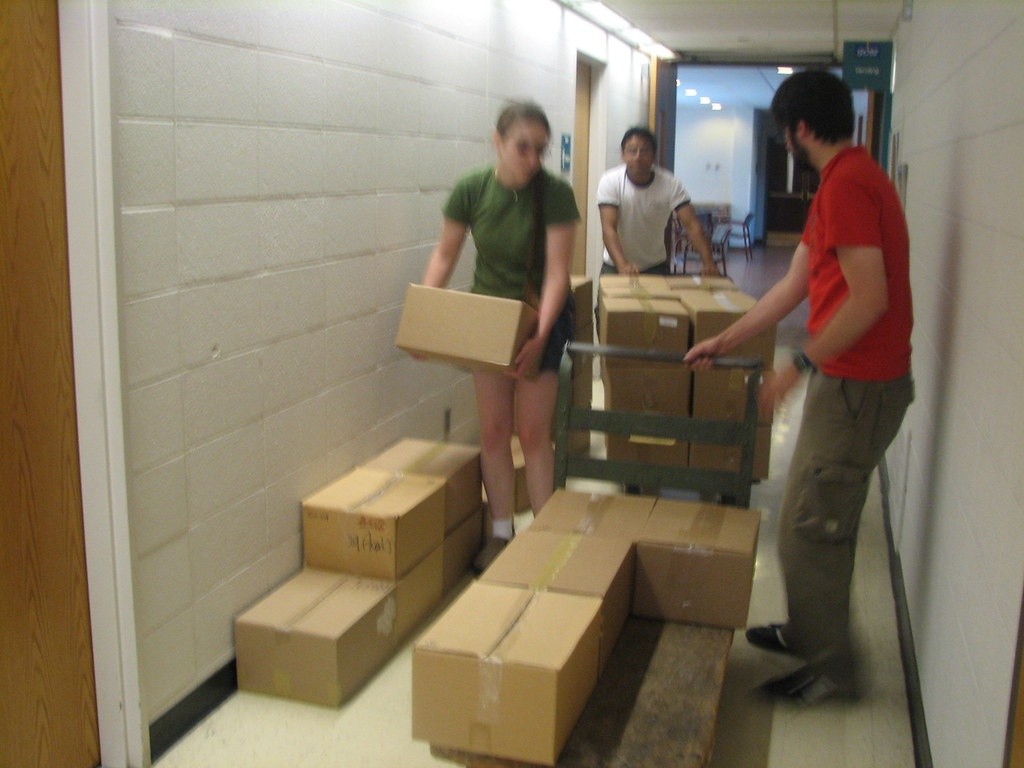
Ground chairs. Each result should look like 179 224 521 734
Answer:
670 210 754 274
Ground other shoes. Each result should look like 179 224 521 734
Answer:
473 531 516 574
746 623 798 654
759 673 810 701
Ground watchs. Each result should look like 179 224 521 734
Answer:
793 351 812 372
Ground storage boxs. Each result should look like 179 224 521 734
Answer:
229 270 773 767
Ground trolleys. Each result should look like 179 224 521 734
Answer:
510 342 765 768
599 272 761 509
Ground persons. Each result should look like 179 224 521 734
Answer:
594 128 720 339
412 104 582 572
682 70 917 705
